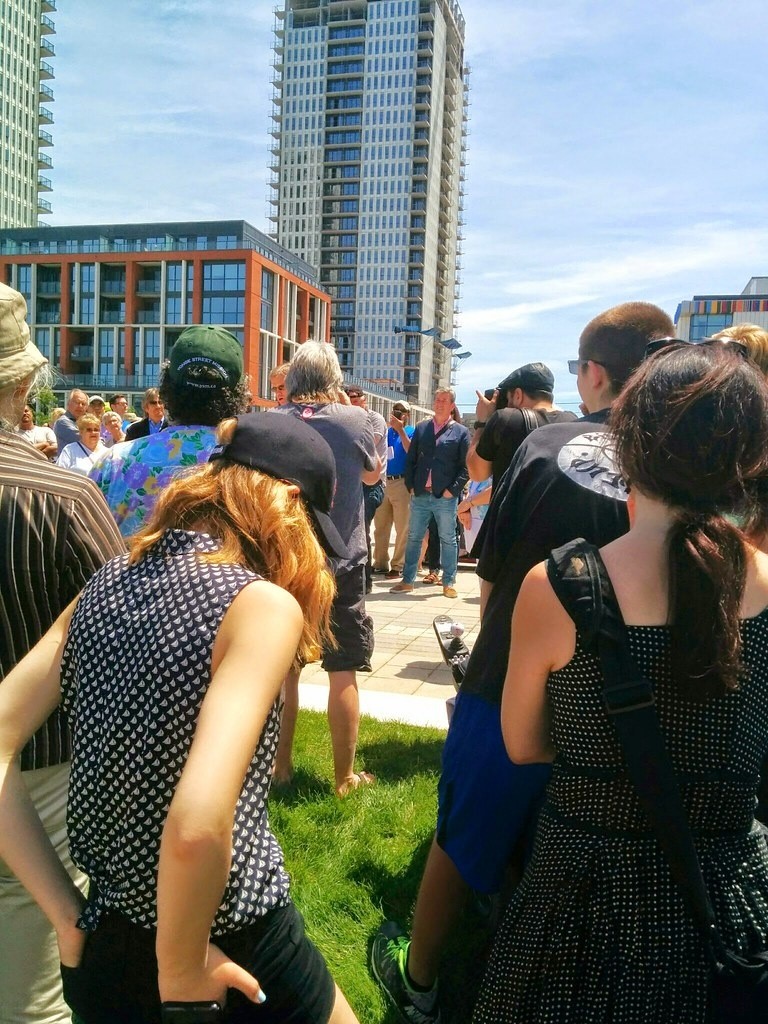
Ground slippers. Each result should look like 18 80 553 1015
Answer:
338 772 379 802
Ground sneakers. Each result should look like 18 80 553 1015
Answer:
390 582 412 593
444 583 457 599
423 572 439 584
371 919 443 1024
371 567 389 576
386 569 405 578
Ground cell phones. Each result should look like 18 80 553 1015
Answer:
394 411 402 419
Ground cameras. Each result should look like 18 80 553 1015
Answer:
485 388 508 411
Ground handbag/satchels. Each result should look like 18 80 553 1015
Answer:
710 948 767 1024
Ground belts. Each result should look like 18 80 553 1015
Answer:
388 474 405 480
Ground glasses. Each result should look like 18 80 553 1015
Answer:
394 408 408 414
567 360 606 374
85 427 99 432
150 400 164 406
645 337 751 362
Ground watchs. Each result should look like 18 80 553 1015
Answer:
474 421 486 430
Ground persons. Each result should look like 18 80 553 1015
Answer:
370 361 589 598
0 408 369 1024
270 337 383 798
0 282 128 1024
473 340 768 1024
708 325 768 556
86 321 253 551
16 359 390 596
370 300 672 1023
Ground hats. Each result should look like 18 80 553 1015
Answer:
207 411 352 560
0 282 49 389
393 400 411 412
345 386 367 398
89 395 105 405
496 362 555 392
165 324 244 391
